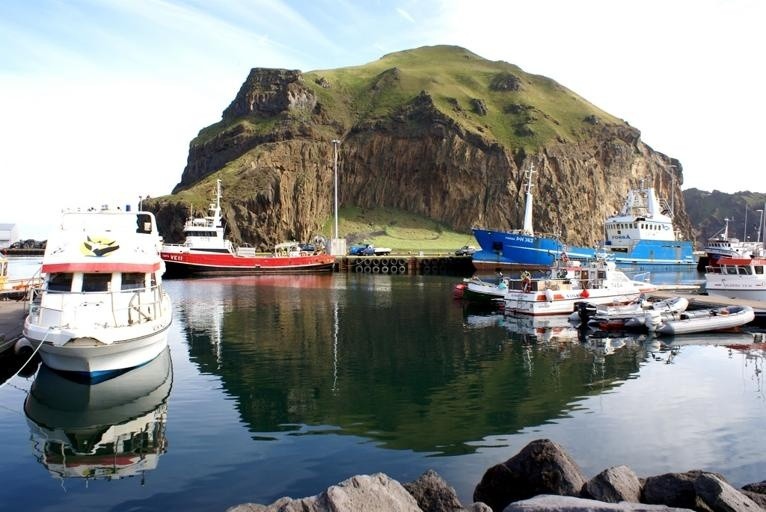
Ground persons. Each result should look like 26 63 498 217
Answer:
498 281 506 289
472 272 479 280
497 272 503 284
521 271 531 292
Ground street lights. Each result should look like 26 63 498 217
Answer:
326 136 346 257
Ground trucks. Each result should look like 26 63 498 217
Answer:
359 243 391 257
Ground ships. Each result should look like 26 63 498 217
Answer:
630 270 699 285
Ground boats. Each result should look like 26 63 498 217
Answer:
498 314 581 377
567 301 639 328
703 208 765 301
179 275 335 310
461 274 510 301
658 333 757 349
652 302 755 338
490 297 506 313
589 331 649 352
13 204 175 375
461 303 501 333
465 157 697 281
17 347 175 493
0 258 43 354
502 242 640 319
158 178 339 272
578 297 689 328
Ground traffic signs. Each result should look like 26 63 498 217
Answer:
329 291 340 395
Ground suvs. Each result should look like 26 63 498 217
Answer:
456 246 477 256
